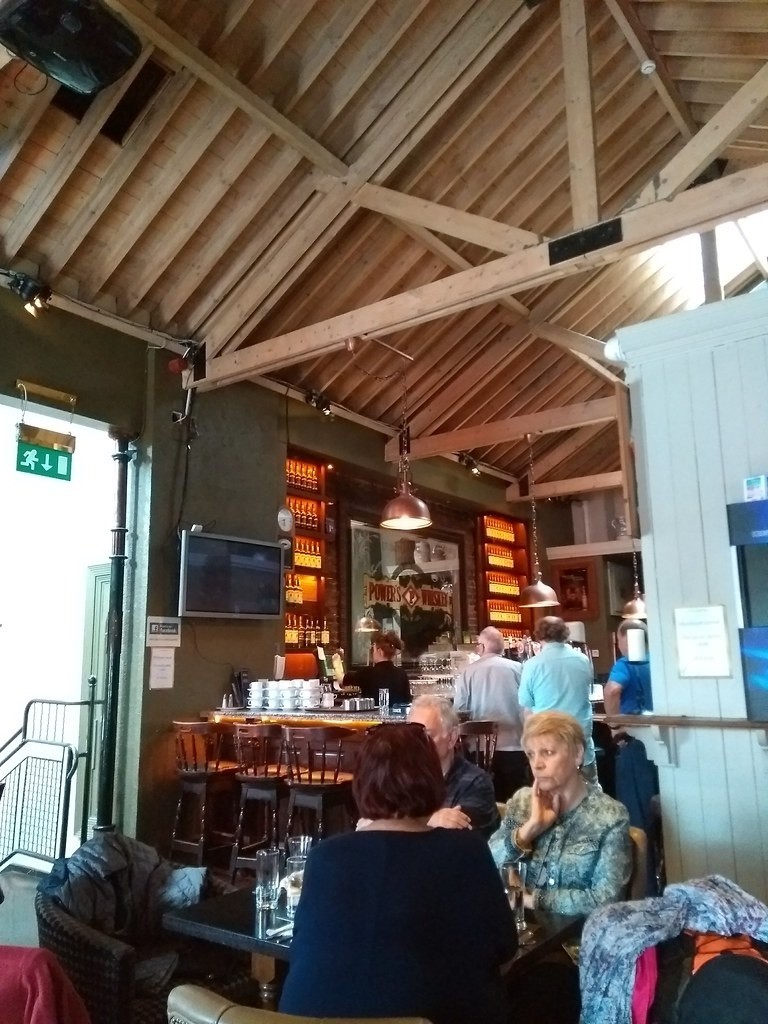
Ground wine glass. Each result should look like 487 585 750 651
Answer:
409 657 456 696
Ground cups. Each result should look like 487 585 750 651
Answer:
255 849 279 910
286 855 308 918
246 700 321 708
344 697 375 710
287 836 313 857
248 690 322 698
499 861 528 929
378 688 390 710
247 678 320 689
323 692 337 708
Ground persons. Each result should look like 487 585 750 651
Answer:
486 709 633 969
407 693 500 830
331 635 410 706
519 615 598 790
604 617 663 896
452 626 534 803
279 721 519 1024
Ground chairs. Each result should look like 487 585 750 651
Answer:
35 801 647 1024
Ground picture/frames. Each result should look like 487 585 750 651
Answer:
550 560 601 622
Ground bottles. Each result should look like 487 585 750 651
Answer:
485 519 515 542
285 614 330 649
506 635 536 663
487 546 514 569
489 602 522 624
284 574 303 605
294 538 321 569
496 626 530 649
222 694 233 707
288 498 318 530
286 460 318 493
488 573 520 596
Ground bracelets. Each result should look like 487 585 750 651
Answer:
610 725 620 729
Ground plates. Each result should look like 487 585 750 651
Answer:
216 705 245 711
245 688 323 690
246 707 318 710
246 697 322 699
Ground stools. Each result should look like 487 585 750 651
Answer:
455 720 499 779
168 720 357 886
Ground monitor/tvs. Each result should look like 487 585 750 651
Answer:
178 531 284 619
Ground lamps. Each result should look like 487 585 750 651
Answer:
9 272 52 309
345 336 432 530
621 537 648 620
304 391 331 416
458 452 479 474
517 433 560 607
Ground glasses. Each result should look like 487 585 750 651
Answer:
475 643 485 648
364 722 429 742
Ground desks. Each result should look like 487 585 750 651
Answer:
161 872 585 979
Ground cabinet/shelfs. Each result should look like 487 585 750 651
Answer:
284 454 337 653
475 512 534 641
